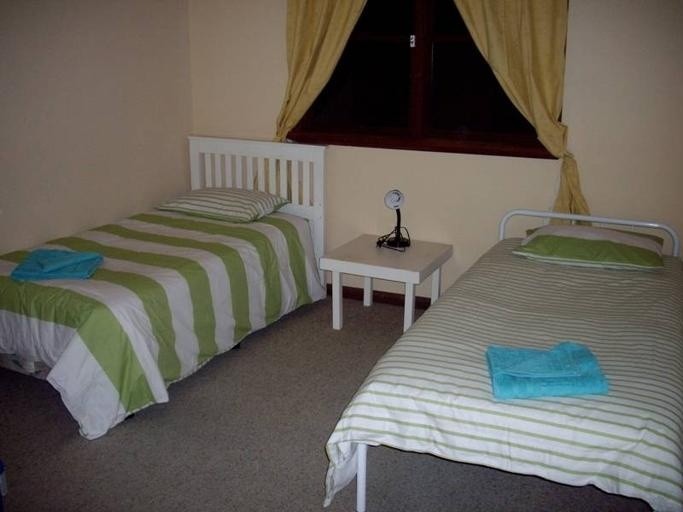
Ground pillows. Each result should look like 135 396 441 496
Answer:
513 224 664 272
156 187 288 224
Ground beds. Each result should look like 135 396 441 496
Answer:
322 209 683 512
0 135 330 440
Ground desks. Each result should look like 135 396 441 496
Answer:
318 234 454 335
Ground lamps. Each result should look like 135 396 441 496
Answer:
377 188 411 253
